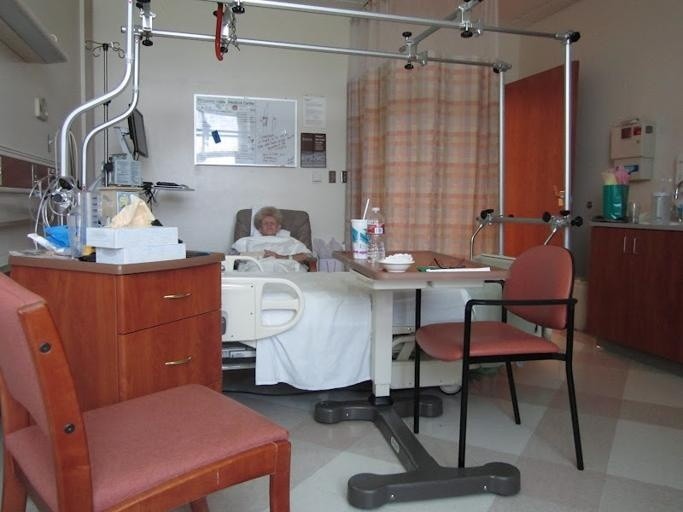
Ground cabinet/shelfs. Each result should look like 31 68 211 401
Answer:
587 226 682 362
6 249 226 425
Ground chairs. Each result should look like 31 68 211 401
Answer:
0 267 292 510
412 243 585 471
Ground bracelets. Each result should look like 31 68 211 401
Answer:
288 254 293 259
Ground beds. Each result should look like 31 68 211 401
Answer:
220 253 540 343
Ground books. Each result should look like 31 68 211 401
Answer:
415 263 491 272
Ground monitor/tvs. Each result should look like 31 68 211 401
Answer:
129 104 148 157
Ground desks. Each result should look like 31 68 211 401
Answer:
313 249 521 510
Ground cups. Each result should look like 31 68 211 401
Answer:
351 219 371 259
628 201 640 224
651 192 672 226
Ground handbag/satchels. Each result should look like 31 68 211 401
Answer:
603 185 629 220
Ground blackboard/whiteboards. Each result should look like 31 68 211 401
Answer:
193 93 299 169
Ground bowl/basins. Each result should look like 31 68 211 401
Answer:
377 261 415 273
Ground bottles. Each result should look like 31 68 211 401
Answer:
366 207 386 264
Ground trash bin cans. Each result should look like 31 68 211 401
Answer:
572 279 588 332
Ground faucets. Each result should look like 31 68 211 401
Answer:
674 181 683 199
